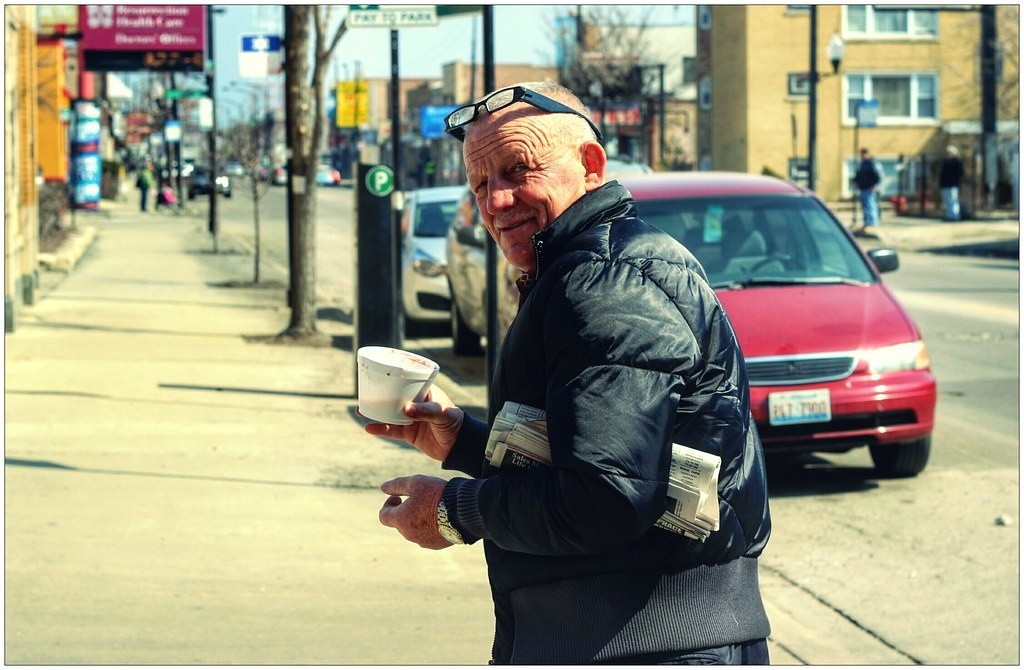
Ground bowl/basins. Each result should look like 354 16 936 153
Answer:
357 345 440 425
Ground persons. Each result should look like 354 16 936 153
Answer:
356 80 770 665
138 164 152 211
156 185 175 207
940 145 965 222
855 147 883 232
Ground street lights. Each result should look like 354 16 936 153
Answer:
810 29 842 194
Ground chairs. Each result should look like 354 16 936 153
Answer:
717 229 786 276
418 207 449 236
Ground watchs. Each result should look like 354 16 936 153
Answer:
437 498 464 545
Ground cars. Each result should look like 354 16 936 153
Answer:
399 187 475 338
495 168 937 479
186 165 233 199
223 160 244 176
271 160 341 185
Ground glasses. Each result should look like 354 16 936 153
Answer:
443 85 605 146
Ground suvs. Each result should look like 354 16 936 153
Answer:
443 156 652 357
